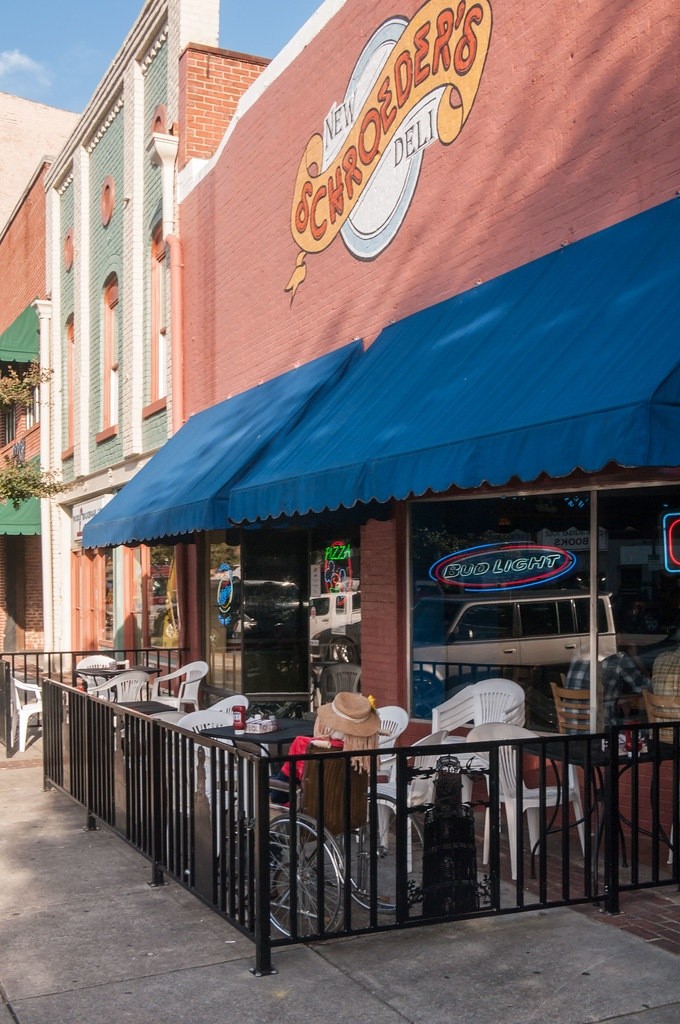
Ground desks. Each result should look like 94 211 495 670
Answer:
113 700 178 757
74 664 163 700
511 732 675 896
198 714 313 826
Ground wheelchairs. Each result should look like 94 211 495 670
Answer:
215 778 424 936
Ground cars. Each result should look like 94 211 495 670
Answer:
209 565 241 588
149 580 309 650
150 565 170 603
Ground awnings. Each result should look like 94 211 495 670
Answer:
0 301 40 363
81 197 680 550
0 463 42 535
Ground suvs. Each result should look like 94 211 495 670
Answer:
409 585 617 720
310 592 362 665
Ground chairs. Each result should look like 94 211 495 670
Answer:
9 676 68 753
75 653 680 882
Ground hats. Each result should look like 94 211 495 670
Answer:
317 693 382 737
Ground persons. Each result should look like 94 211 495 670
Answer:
562 621 649 754
651 648 680 746
271 691 377 835
